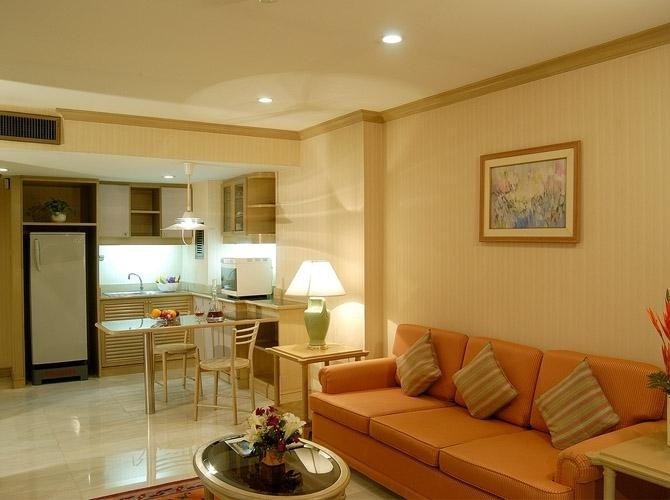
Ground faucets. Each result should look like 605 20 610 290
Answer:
129 320 144 330
128 273 143 290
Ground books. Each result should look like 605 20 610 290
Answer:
225 434 304 458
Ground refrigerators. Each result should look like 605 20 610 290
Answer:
29 232 87 365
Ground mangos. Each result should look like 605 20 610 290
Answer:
151 309 162 318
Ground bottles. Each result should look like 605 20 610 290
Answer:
206 278 225 323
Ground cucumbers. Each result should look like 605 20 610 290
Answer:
160 276 167 285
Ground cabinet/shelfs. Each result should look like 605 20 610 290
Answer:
99 295 193 377
192 294 310 405
96 180 192 245
222 172 276 244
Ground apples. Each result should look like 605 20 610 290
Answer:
161 310 168 315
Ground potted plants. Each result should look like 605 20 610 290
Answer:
41 199 71 222
647 288 670 448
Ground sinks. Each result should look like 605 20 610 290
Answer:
103 292 145 296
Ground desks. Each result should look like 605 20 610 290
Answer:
265 343 369 422
592 431 670 500
95 314 279 414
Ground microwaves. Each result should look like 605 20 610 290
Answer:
218 256 274 297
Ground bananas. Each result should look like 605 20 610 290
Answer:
168 310 176 318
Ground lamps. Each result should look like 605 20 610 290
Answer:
160 163 212 247
285 260 346 350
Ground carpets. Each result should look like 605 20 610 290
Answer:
90 477 221 500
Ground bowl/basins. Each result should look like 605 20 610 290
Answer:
150 321 182 329
146 313 180 321
156 282 178 292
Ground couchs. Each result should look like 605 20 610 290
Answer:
308 324 667 500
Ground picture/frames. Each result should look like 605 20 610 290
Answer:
479 140 581 245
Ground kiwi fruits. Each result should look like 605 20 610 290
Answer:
176 310 179 316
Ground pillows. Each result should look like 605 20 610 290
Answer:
392 322 667 450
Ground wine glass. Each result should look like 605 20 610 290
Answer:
193 304 205 325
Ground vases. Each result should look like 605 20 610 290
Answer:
259 446 286 465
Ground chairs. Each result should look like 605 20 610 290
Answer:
193 322 260 425
145 311 204 402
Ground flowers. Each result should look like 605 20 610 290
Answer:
243 407 306 461
